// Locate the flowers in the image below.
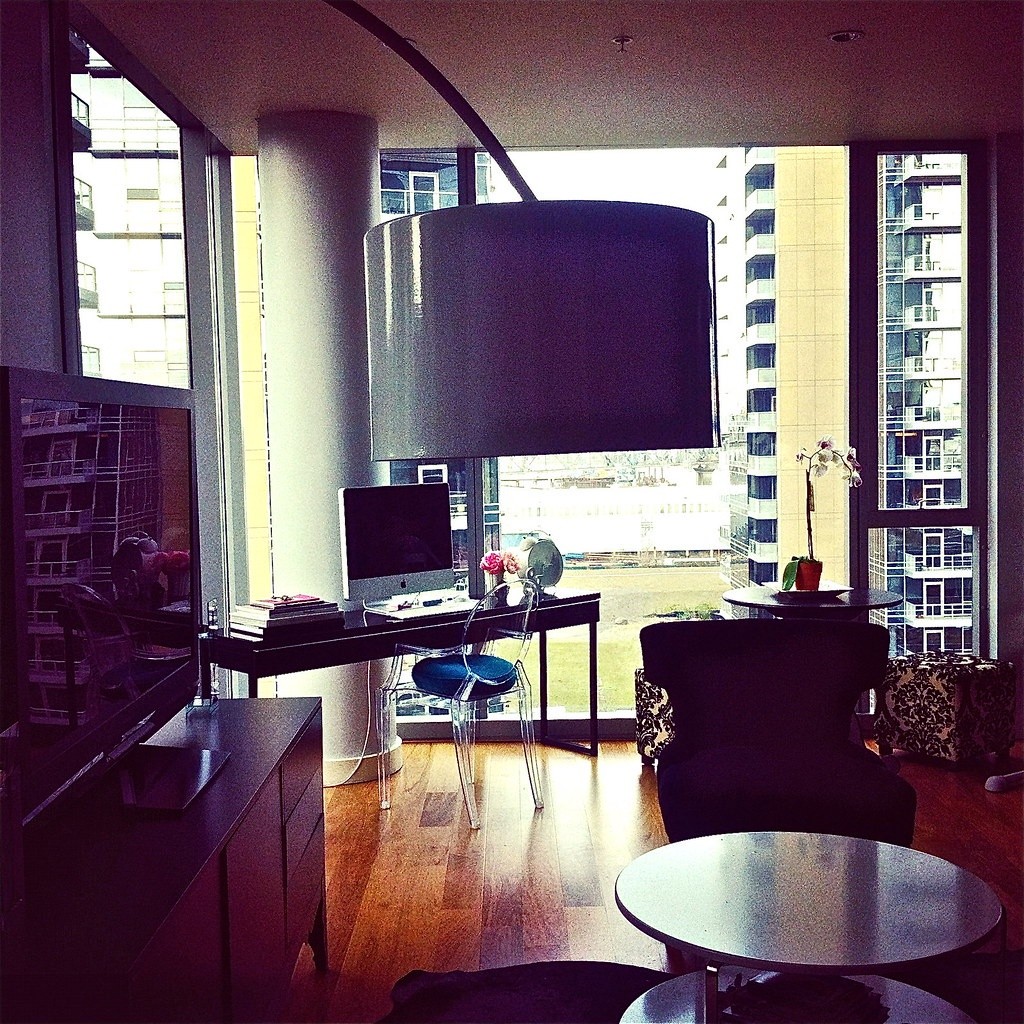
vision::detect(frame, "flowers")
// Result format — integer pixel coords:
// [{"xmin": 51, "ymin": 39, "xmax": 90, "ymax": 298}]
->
[
  {"xmin": 781, "ymin": 435, "xmax": 863, "ymax": 590},
  {"xmin": 479, "ymin": 551, "xmax": 520, "ymax": 575}
]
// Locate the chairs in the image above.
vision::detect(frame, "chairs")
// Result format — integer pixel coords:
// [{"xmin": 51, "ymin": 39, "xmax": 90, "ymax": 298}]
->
[
  {"xmin": 635, "ymin": 617, "xmax": 918, "ymax": 841},
  {"xmin": 373, "ymin": 577, "xmax": 545, "ymax": 831}
]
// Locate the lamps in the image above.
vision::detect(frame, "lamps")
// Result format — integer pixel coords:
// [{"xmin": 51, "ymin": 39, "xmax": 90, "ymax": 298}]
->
[{"xmin": 332, "ymin": 1, "xmax": 720, "ymax": 464}]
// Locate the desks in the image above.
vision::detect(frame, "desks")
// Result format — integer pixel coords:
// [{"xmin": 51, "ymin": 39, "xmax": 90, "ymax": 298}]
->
[
  {"xmin": 722, "ymin": 587, "xmax": 904, "ymax": 618},
  {"xmin": 614, "ymin": 832, "xmax": 1003, "ymax": 1024},
  {"xmin": 198, "ymin": 589, "xmax": 601, "ymax": 756}
]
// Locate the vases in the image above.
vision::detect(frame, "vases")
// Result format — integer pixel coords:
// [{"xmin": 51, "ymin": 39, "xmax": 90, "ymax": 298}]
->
[
  {"xmin": 796, "ymin": 561, "xmax": 822, "ymax": 590},
  {"xmin": 493, "ymin": 575, "xmax": 510, "ymax": 607}
]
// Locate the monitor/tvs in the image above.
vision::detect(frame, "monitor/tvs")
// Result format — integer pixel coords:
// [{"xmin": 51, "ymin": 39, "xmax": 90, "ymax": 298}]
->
[
  {"xmin": 338, "ymin": 483, "xmax": 454, "ymax": 612},
  {"xmin": 0, "ymin": 367, "xmax": 233, "ymax": 829}
]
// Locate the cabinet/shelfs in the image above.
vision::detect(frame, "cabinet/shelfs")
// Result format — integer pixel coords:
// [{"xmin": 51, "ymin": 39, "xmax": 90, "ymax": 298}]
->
[{"xmin": 0, "ymin": 698, "xmax": 327, "ymax": 1024}]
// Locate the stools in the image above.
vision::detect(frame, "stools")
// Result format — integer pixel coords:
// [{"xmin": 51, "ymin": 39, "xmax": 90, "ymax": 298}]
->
[
  {"xmin": 876, "ymin": 651, "xmax": 1017, "ymax": 769},
  {"xmin": 635, "ymin": 669, "xmax": 676, "ymax": 762}
]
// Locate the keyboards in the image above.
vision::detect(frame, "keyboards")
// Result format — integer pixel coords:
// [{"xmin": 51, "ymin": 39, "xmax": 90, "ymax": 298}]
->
[{"xmin": 389, "ymin": 601, "xmax": 483, "ymax": 621}]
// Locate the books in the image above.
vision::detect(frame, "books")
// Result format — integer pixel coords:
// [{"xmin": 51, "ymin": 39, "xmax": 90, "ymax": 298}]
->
[{"xmin": 229, "ymin": 593, "xmax": 346, "ymax": 639}]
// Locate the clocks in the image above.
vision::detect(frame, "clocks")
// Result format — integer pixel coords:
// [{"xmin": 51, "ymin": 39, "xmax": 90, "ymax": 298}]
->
[{"xmin": 518, "ymin": 530, "xmax": 563, "ymax": 588}]
